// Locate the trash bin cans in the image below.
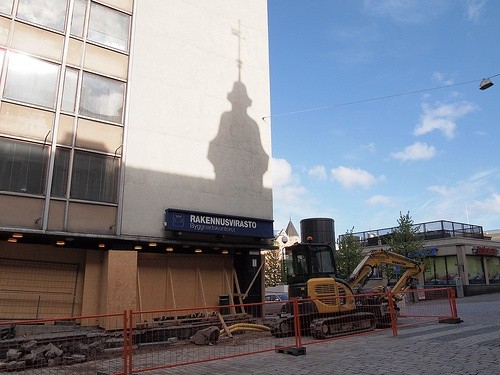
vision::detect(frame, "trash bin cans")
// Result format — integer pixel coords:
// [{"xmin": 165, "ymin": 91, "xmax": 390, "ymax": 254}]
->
[{"xmin": 218, "ymin": 293, "xmax": 249, "ymax": 314}]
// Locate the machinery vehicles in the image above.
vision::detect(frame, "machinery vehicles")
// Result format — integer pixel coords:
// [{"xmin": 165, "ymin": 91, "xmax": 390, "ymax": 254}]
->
[{"xmin": 268, "ymin": 236, "xmax": 425, "ymax": 339}]
